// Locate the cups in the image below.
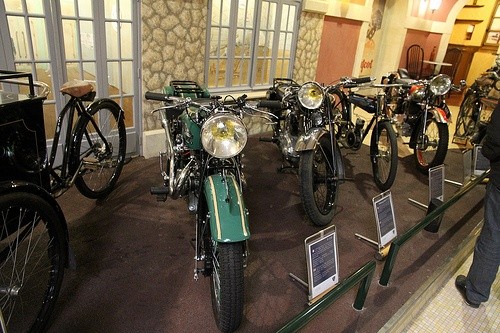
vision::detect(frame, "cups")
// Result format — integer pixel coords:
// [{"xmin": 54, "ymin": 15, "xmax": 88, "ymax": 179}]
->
[{"xmin": 464, "ymin": 24, "xmax": 475, "ymax": 40}]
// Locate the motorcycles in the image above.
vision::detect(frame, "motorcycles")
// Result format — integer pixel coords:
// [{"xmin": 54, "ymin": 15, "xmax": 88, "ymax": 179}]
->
[
  {"xmin": 257, "ymin": 76, "xmax": 376, "ymax": 227},
  {"xmin": 451, "ymin": 52, "xmax": 500, "ymax": 146},
  {"xmin": 144, "ymin": 79, "xmax": 286, "ymax": 333},
  {"xmin": 381, "ymin": 67, "xmax": 467, "ymax": 177}
]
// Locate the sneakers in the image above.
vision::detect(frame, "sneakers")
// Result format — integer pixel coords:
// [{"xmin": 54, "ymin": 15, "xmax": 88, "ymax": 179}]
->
[{"xmin": 455, "ymin": 275, "xmax": 480, "ymax": 308}]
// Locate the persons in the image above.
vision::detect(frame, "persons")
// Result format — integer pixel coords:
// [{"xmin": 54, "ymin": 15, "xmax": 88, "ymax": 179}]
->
[{"xmin": 454, "ymin": 100, "xmax": 500, "ymax": 308}]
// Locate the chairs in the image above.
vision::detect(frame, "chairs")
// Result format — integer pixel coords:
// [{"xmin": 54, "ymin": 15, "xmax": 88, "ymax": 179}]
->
[{"xmin": 406, "ymin": 45, "xmax": 424, "ymax": 80}]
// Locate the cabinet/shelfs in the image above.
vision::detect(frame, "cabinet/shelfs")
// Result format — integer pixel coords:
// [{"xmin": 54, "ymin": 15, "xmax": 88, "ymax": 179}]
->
[
  {"xmin": 455, "ymin": 4, "xmax": 484, "ymax": 22},
  {"xmin": 439, "ymin": 44, "xmax": 482, "ymax": 106}
]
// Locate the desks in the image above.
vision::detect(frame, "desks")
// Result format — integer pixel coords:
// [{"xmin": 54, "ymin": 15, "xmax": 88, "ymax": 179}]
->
[
  {"xmin": 421, "ymin": 59, "xmax": 452, "ymax": 76},
  {"xmin": 0, "ymin": 69, "xmax": 51, "ymax": 241}
]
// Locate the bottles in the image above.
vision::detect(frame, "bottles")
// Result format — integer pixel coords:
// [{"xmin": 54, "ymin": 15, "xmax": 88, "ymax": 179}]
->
[{"xmin": 429, "ymin": 46, "xmax": 437, "ymax": 61}]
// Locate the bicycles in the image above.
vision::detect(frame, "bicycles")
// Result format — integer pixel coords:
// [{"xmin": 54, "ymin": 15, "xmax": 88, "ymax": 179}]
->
[
  {"xmin": 321, "ymin": 74, "xmax": 413, "ymax": 193},
  {"xmin": 0, "ymin": 78, "xmax": 140, "ymax": 333}
]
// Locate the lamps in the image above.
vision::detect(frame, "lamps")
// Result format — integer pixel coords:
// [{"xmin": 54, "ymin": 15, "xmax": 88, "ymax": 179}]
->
[{"xmin": 430, "ymin": 0, "xmax": 442, "ymax": 15}]
